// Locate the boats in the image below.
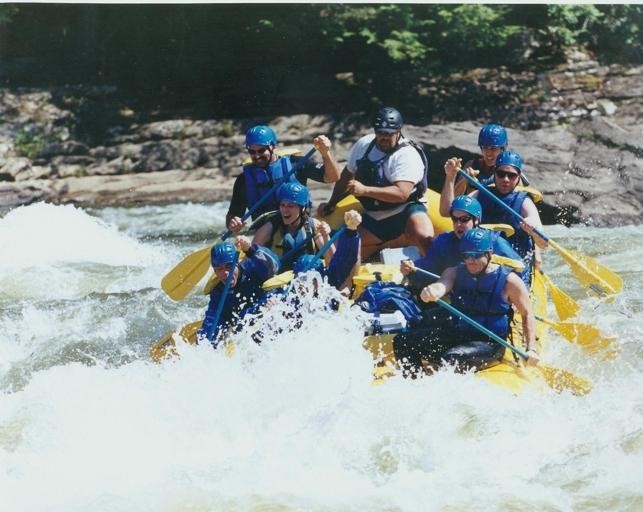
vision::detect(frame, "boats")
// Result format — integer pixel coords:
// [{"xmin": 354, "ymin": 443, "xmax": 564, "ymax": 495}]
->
[{"xmin": 147, "ymin": 186, "xmax": 550, "ymax": 403}]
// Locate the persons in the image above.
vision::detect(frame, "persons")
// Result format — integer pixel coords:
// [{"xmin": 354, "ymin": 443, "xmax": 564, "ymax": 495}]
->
[
  {"xmin": 225, "ymin": 124, "xmax": 342, "ymax": 233},
  {"xmin": 454, "ymin": 121, "xmax": 530, "ymax": 197},
  {"xmin": 317, "ymin": 105, "xmax": 434, "ymax": 262},
  {"xmin": 195, "ymin": 240, "xmax": 282, "ymax": 346},
  {"xmin": 294, "ymin": 209, "xmax": 363, "ymax": 289},
  {"xmin": 393, "ymin": 228, "xmax": 540, "ymax": 377},
  {"xmin": 440, "ymin": 149, "xmax": 550, "ymax": 291},
  {"xmin": 400, "ymin": 195, "xmax": 531, "ymax": 308},
  {"xmin": 251, "ymin": 182, "xmax": 336, "ymax": 269}
]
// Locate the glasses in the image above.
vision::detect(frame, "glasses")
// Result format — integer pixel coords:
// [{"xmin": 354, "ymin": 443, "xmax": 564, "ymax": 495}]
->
[
  {"xmin": 451, "ymin": 216, "xmax": 472, "ymax": 222},
  {"xmin": 462, "ymin": 253, "xmax": 483, "ymax": 259},
  {"xmin": 497, "ymin": 170, "xmax": 517, "ymax": 181},
  {"xmin": 248, "ymin": 148, "xmax": 266, "ymax": 154}
]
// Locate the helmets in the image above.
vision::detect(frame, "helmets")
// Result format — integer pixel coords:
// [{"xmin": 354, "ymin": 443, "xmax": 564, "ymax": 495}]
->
[
  {"xmin": 211, "ymin": 242, "xmax": 235, "ymax": 267},
  {"xmin": 478, "ymin": 124, "xmax": 521, "ymax": 171},
  {"xmin": 293, "ymin": 254, "xmax": 323, "ymax": 276},
  {"xmin": 459, "ymin": 229, "xmax": 493, "ymax": 253},
  {"xmin": 246, "ymin": 125, "xmax": 278, "ymax": 146},
  {"xmin": 372, "ymin": 106, "xmax": 403, "ymax": 133},
  {"xmin": 450, "ymin": 195, "xmax": 482, "ymax": 223},
  {"xmin": 276, "ymin": 182, "xmax": 310, "ymax": 206}
]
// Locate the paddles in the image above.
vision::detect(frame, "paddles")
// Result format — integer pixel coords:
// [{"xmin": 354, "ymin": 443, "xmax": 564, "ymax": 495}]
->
[
  {"xmin": 161, "ymin": 146, "xmax": 320, "ymax": 301},
  {"xmin": 433, "ymin": 296, "xmax": 592, "ymax": 397},
  {"xmin": 151, "ymin": 321, "xmax": 206, "ymax": 367},
  {"xmin": 453, "ymin": 166, "xmax": 624, "ymax": 304},
  {"xmin": 535, "ymin": 261, "xmax": 583, "ymax": 322},
  {"xmin": 533, "ymin": 314, "xmax": 619, "ymax": 364}
]
